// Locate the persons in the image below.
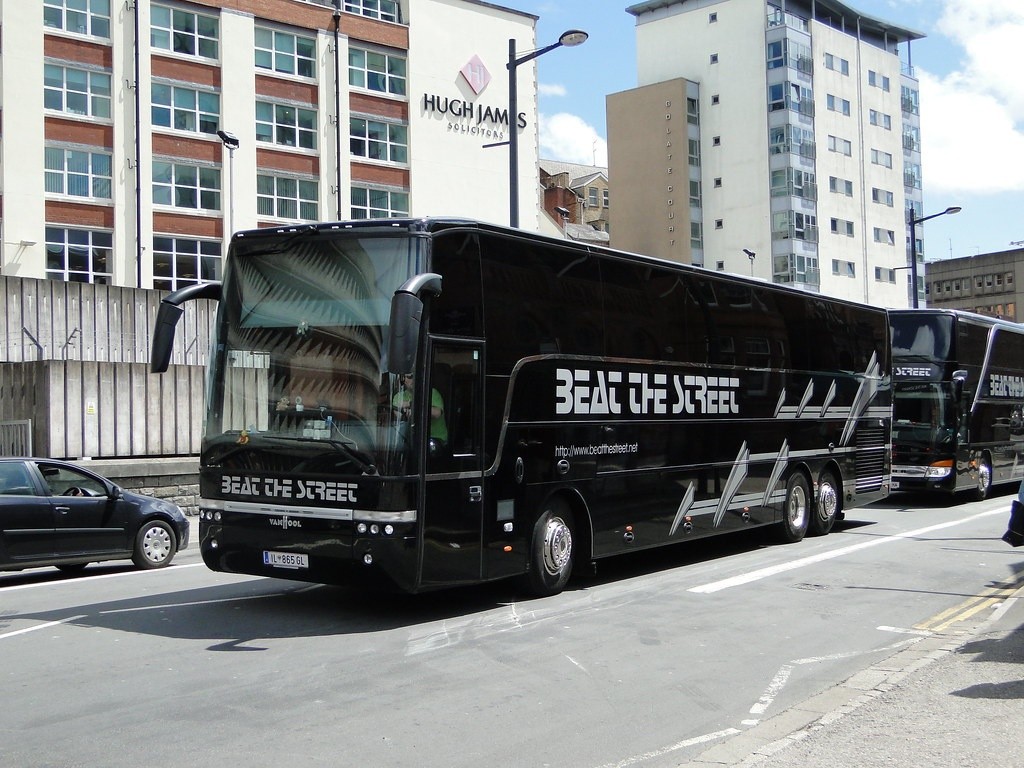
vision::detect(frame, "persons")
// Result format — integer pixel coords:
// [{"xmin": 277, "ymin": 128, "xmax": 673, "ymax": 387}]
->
[{"xmin": 393, "ymin": 372, "xmax": 449, "ymax": 472}]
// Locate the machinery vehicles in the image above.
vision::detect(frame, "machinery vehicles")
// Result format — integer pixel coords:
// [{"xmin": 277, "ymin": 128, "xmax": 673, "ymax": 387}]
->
[{"xmin": 888, "ymin": 309, "xmax": 1024, "ymax": 499}]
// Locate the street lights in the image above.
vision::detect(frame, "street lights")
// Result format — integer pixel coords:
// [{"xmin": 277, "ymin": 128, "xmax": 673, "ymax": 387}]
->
[
  {"xmin": 507, "ymin": 32, "xmax": 586, "ymax": 229},
  {"xmin": 910, "ymin": 207, "xmax": 959, "ymax": 308}
]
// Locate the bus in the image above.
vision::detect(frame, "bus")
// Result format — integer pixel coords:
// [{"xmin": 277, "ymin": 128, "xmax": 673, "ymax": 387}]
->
[{"xmin": 151, "ymin": 217, "xmax": 894, "ymax": 598}]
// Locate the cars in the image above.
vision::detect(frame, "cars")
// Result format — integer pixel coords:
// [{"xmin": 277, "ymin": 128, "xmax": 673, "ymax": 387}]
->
[{"xmin": 1, "ymin": 457, "xmax": 189, "ymax": 571}]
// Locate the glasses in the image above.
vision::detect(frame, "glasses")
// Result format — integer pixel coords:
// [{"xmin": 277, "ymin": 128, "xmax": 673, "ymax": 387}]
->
[{"xmin": 404, "ymin": 374, "xmax": 413, "ymax": 379}]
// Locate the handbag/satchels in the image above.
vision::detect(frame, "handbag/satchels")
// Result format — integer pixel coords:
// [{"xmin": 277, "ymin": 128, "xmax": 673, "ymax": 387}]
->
[{"xmin": 1002, "ymin": 500, "xmax": 1024, "ymax": 547}]
